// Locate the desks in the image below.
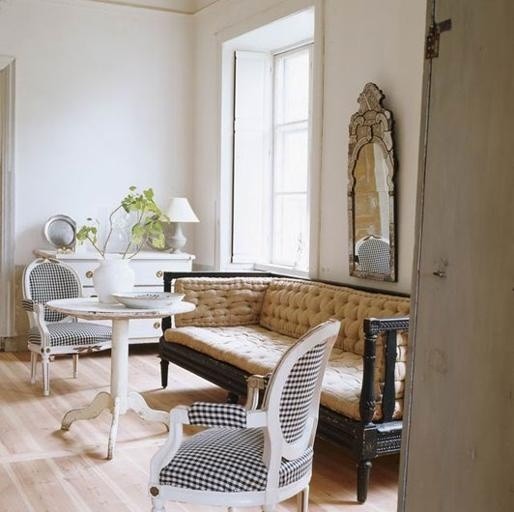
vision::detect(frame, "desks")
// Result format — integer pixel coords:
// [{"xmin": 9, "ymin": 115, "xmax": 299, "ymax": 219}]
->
[{"xmin": 46, "ymin": 297, "xmax": 195, "ymax": 461}]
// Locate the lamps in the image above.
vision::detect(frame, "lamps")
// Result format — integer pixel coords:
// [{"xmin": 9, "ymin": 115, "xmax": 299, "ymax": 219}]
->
[{"xmin": 160, "ymin": 197, "xmax": 200, "ymax": 255}]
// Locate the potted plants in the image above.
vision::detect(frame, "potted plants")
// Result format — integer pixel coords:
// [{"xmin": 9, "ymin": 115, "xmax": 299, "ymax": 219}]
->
[{"xmin": 76, "ymin": 186, "xmax": 170, "ymax": 304}]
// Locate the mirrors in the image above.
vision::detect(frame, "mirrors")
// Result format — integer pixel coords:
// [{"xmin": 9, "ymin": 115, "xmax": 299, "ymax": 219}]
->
[{"xmin": 347, "ymin": 82, "xmax": 398, "ymax": 283}]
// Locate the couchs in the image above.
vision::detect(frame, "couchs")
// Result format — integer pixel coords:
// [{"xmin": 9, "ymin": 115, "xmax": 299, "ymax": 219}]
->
[{"xmin": 158, "ymin": 271, "xmax": 410, "ymax": 505}]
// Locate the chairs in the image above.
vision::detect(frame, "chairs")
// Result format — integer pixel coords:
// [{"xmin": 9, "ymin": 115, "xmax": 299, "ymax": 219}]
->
[
  {"xmin": 21, "ymin": 259, "xmax": 112, "ymax": 397},
  {"xmin": 149, "ymin": 320, "xmax": 342, "ymax": 512},
  {"xmin": 354, "ymin": 234, "xmax": 390, "ymax": 273}
]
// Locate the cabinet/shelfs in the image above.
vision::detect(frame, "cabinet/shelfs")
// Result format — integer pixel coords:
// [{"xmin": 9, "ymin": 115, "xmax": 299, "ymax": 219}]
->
[{"xmin": 34, "ymin": 248, "xmax": 195, "ymax": 344}]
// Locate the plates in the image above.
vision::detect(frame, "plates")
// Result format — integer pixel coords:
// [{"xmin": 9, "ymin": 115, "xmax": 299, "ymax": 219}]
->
[{"xmin": 111, "ymin": 291, "xmax": 186, "ymax": 310}]
